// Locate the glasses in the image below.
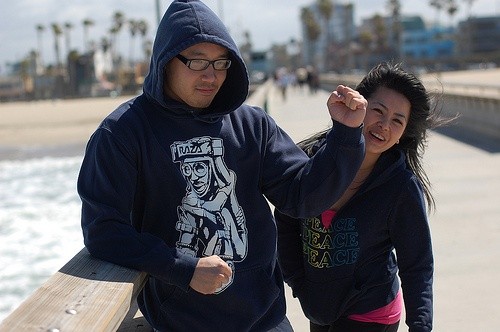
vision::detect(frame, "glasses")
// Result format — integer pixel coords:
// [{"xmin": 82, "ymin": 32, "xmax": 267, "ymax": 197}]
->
[{"xmin": 176, "ymin": 54, "xmax": 232, "ymax": 71}]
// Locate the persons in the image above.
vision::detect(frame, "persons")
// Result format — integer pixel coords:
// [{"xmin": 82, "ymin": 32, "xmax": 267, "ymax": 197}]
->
[
  {"xmin": 275, "ymin": 65, "xmax": 320, "ymax": 99},
  {"xmin": 273, "ymin": 60, "xmax": 463, "ymax": 332},
  {"xmin": 77, "ymin": 0, "xmax": 368, "ymax": 332}
]
